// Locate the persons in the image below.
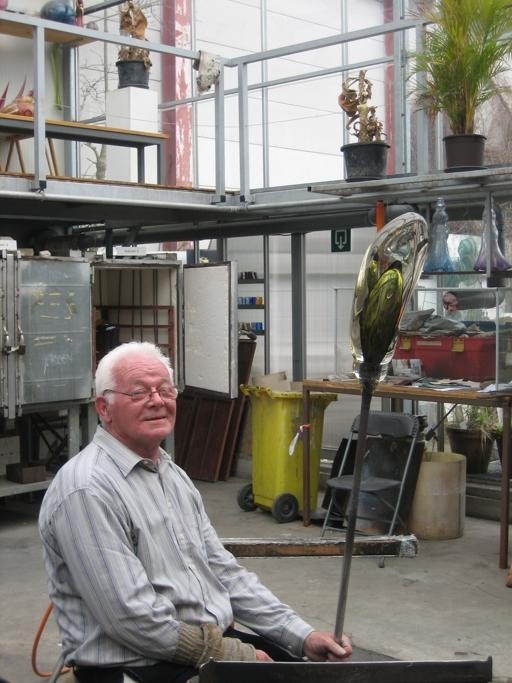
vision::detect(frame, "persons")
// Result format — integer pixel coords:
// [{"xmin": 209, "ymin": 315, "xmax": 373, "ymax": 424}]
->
[{"xmin": 38, "ymin": 341, "xmax": 353, "ymax": 683}]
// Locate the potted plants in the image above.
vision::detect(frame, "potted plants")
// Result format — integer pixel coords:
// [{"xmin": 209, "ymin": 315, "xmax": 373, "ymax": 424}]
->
[
  {"xmin": 403, "ymin": 0, "xmax": 511, "ymax": 173},
  {"xmin": 117, "ymin": 0, "xmax": 152, "ymax": 88},
  {"xmin": 335, "ymin": 69, "xmax": 389, "ymax": 180},
  {"xmin": 445, "ymin": 408, "xmax": 505, "ymax": 475}
]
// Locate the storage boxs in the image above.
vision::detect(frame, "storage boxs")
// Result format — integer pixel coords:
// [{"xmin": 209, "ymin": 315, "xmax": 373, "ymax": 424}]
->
[{"xmin": 391, "ymin": 332, "xmax": 507, "ymax": 385}]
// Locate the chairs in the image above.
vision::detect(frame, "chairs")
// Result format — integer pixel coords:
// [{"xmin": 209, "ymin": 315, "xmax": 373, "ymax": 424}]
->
[{"xmin": 319, "ymin": 411, "xmax": 419, "ymax": 567}]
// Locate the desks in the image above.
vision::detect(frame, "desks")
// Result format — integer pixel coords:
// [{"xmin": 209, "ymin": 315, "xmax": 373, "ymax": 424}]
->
[
  {"xmin": 296, "ymin": 380, "xmax": 512, "ymax": 572},
  {"xmin": 1, "ymin": 111, "xmax": 169, "ymax": 185}
]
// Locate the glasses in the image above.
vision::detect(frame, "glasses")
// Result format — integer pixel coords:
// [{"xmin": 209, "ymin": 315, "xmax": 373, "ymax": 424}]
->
[{"xmin": 103, "ymin": 386, "xmax": 178, "ymax": 402}]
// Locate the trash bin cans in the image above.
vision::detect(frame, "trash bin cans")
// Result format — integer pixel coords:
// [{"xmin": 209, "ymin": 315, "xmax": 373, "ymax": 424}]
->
[{"xmin": 237, "ymin": 384, "xmax": 338, "ymax": 522}]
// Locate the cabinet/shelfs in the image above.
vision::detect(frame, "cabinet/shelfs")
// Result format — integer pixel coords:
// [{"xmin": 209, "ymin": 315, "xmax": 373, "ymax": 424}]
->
[{"xmin": 237, "ymin": 276, "xmax": 266, "ymax": 336}]
